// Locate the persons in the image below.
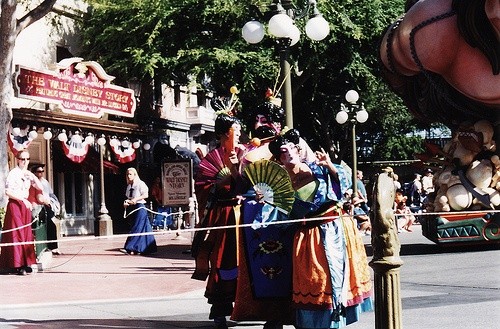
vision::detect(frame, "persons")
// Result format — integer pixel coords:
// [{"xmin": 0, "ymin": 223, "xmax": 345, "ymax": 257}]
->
[
  {"xmin": 191, "ymin": 98, "xmax": 264, "ymax": 329},
  {"xmin": 256, "ymin": 128, "xmax": 376, "ymax": 329},
  {"xmin": 151, "ymin": 177, "xmax": 189, "ymax": 231},
  {"xmin": 119, "ymin": 168, "xmax": 159, "ymax": 255},
  {"xmin": 343, "ymin": 167, "xmax": 435, "ymax": 233},
  {"xmin": 0, "ymin": 149, "xmax": 51, "ymax": 275},
  {"xmin": 230, "ymin": 104, "xmax": 318, "ymax": 329},
  {"xmin": 27, "ymin": 164, "xmax": 65, "ymax": 255}
]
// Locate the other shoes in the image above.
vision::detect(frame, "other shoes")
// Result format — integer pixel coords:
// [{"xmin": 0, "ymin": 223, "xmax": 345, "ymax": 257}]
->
[
  {"xmin": 18, "ymin": 268, "xmax": 27, "ymax": 275},
  {"xmin": 213, "ymin": 316, "xmax": 229, "ymax": 329},
  {"xmin": 120, "ymin": 249, "xmax": 130, "ymax": 255},
  {"xmin": 25, "ymin": 267, "xmax": 32, "ymax": 273}
]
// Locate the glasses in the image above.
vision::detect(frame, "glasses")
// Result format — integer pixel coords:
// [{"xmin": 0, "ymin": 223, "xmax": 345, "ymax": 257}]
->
[
  {"xmin": 18, "ymin": 158, "xmax": 30, "ymax": 160},
  {"xmin": 35, "ymin": 170, "xmax": 44, "ymax": 173}
]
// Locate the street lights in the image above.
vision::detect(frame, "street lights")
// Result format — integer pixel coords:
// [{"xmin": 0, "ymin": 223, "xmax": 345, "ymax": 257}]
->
[
  {"xmin": 335, "ymin": 88, "xmax": 369, "ymax": 197},
  {"xmin": 241, "ymin": 0, "xmax": 331, "ymax": 130}
]
